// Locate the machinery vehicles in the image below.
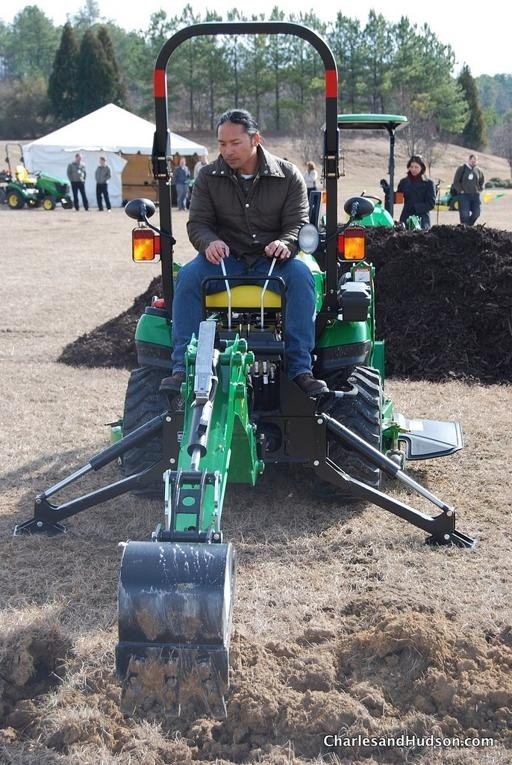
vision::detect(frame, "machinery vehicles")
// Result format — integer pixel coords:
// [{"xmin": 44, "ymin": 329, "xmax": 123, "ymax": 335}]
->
[
  {"xmin": 427, "ymin": 176, "xmax": 461, "ymax": 211},
  {"xmin": 0, "ymin": 170, "xmax": 13, "ymax": 205},
  {"xmin": 10, "ymin": 18, "xmax": 479, "ymax": 722},
  {"xmin": 309, "ymin": 111, "xmax": 423, "ymax": 232}
]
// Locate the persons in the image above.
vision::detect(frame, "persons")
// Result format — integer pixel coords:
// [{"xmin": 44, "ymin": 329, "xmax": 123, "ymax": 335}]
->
[
  {"xmin": 159, "ymin": 109, "xmax": 331, "ymax": 396},
  {"xmin": 397, "ymin": 155, "xmax": 436, "ymax": 232},
  {"xmin": 173, "ymin": 157, "xmax": 191, "ymax": 211},
  {"xmin": 194, "ymin": 155, "xmax": 210, "ymax": 182},
  {"xmin": 94, "ymin": 157, "xmax": 112, "ymax": 213},
  {"xmin": 303, "ymin": 161, "xmax": 316, "ymax": 199},
  {"xmin": 284, "ymin": 158, "xmax": 288, "ymax": 161},
  {"xmin": 67, "ymin": 153, "xmax": 90, "ymax": 211},
  {"xmin": 453, "ymin": 154, "xmax": 484, "ymax": 225}
]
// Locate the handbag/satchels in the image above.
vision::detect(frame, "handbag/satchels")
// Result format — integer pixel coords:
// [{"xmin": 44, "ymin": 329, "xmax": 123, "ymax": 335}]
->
[{"xmin": 449, "ymin": 183, "xmax": 459, "ymax": 197}]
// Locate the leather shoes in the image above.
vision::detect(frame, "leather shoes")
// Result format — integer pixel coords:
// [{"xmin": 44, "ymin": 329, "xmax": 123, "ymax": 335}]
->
[
  {"xmin": 159, "ymin": 371, "xmax": 186, "ymax": 392},
  {"xmin": 294, "ymin": 374, "xmax": 329, "ymax": 396}
]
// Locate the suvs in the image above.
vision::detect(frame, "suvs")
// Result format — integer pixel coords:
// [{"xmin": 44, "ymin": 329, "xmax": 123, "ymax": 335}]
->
[{"xmin": 5, "ymin": 142, "xmax": 73, "ymax": 210}]
